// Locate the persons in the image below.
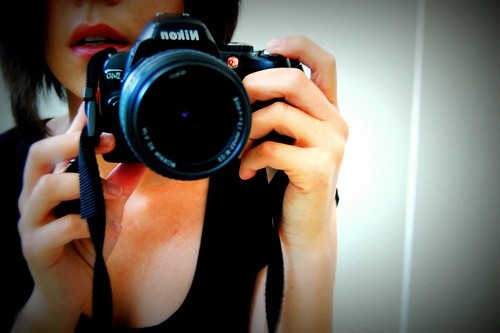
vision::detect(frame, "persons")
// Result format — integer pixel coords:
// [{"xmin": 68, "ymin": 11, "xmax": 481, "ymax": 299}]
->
[{"xmin": 0, "ymin": 0, "xmax": 350, "ymax": 333}]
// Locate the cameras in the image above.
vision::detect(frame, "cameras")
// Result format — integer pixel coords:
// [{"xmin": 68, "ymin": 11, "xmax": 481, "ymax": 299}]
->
[{"xmin": 98, "ymin": 13, "xmax": 301, "ymax": 181}]
